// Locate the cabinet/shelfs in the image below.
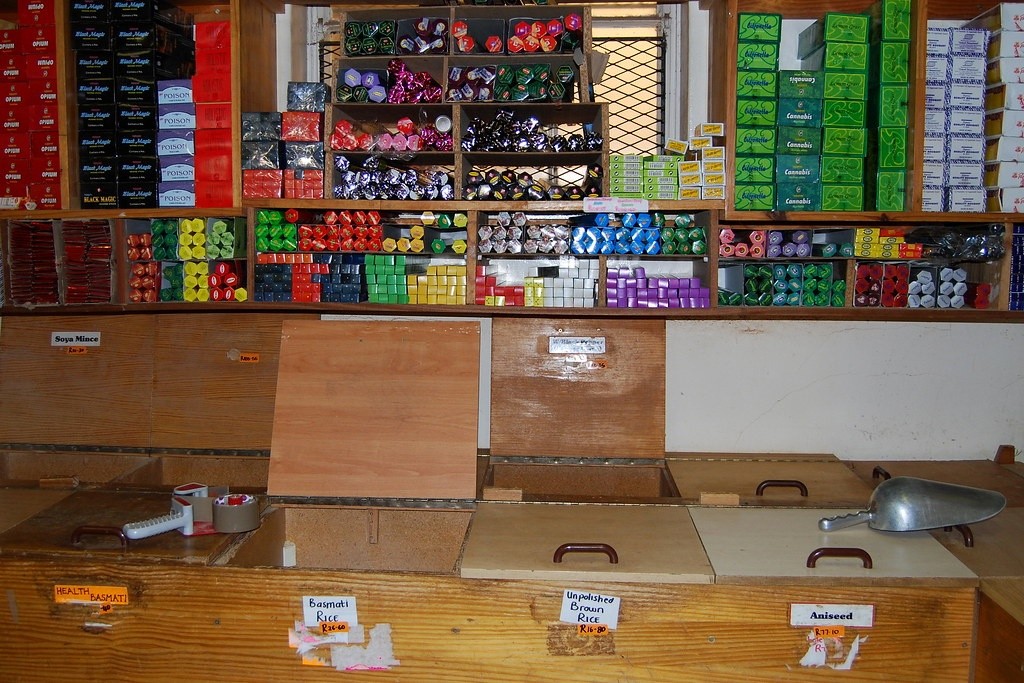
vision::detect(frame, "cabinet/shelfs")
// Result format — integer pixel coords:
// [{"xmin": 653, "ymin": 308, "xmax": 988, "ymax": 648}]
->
[
  {"xmin": 709, "ymin": 0, "xmax": 1024, "ymax": 325},
  {"xmin": 327, "ymin": 6, "xmax": 608, "ymax": 203},
  {"xmin": 240, "ymin": 190, "xmax": 728, "ymax": 319},
  {"xmin": 0, "ymin": 0, "xmax": 245, "ymax": 310}
]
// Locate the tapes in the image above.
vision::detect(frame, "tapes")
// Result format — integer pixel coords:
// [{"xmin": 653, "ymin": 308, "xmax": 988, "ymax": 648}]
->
[{"xmin": 212, "ymin": 494, "xmax": 260, "ymax": 533}]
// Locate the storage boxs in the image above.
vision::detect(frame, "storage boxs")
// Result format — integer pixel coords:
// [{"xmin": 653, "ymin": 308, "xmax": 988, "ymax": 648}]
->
[{"xmin": 1, "ymin": 1, "xmax": 1023, "ymax": 308}]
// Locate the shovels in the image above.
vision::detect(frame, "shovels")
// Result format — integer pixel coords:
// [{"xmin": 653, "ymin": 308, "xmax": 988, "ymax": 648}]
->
[{"xmin": 818, "ymin": 476, "xmax": 1006, "ymax": 532}]
